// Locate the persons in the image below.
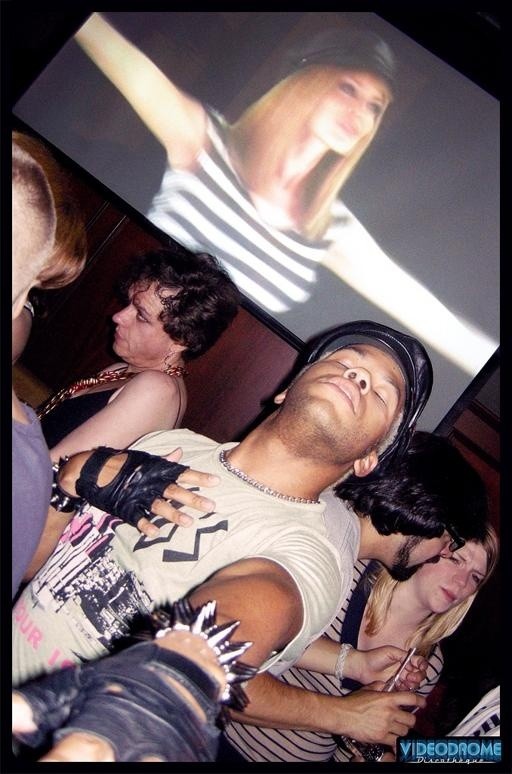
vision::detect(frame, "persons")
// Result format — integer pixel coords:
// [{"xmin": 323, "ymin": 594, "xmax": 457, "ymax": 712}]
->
[
  {"xmin": 9, "ymin": 127, "xmax": 500, "ymax": 762},
  {"xmin": 76, "ymin": 6, "xmax": 500, "ymax": 379}
]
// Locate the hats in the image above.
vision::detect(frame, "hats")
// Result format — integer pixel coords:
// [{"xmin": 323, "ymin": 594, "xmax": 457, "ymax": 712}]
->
[{"xmin": 297, "ymin": 27, "xmax": 402, "ymax": 102}]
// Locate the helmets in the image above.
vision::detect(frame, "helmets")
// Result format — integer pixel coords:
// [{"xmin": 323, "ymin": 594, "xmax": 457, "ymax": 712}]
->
[{"xmin": 307, "ymin": 321, "xmax": 433, "ymax": 463}]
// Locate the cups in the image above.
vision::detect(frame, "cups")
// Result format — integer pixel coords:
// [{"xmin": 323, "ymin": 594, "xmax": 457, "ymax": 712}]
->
[{"xmin": 341, "ymin": 677, "xmax": 419, "ymax": 757}]
